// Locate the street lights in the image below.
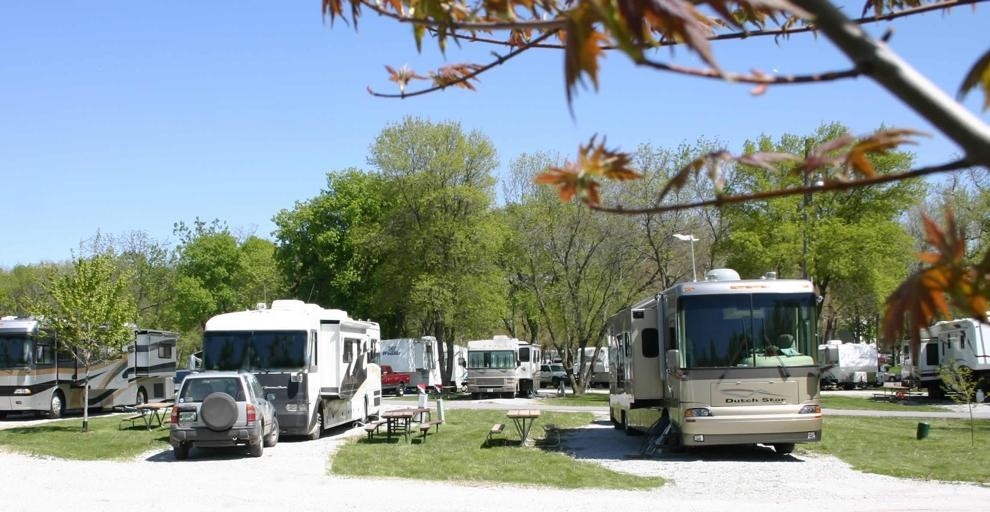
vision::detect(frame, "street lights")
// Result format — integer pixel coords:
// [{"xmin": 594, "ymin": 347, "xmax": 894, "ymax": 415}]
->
[
  {"xmin": 672, "ymin": 230, "xmax": 701, "ymax": 281},
  {"xmin": 802, "ymin": 173, "xmax": 828, "ymax": 277}
]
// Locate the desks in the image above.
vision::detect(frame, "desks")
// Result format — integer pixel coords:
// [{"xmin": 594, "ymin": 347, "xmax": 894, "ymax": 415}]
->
[
  {"xmin": 381, "ymin": 406, "xmax": 434, "ymax": 441},
  {"xmin": 880, "ymin": 382, "xmax": 910, "ymax": 404},
  {"xmin": 133, "ymin": 402, "xmax": 176, "ymax": 432},
  {"xmin": 507, "ymin": 408, "xmax": 541, "ymax": 445}
]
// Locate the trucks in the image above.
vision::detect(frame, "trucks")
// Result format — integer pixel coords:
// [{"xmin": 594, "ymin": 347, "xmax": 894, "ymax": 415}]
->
[{"xmin": 818, "ymin": 313, "xmax": 990, "ymax": 405}]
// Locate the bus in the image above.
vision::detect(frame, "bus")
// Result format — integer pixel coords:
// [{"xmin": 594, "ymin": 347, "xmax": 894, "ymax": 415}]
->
[
  {"xmin": 0, "ymin": 309, "xmax": 186, "ymax": 424},
  {"xmin": 608, "ymin": 268, "xmax": 824, "ymax": 454},
  {"xmin": 381, "ymin": 335, "xmax": 609, "ymax": 397},
  {"xmin": 199, "ymin": 298, "xmax": 381, "ymax": 442}
]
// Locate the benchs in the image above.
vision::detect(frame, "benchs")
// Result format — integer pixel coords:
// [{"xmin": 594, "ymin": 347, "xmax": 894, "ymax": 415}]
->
[
  {"xmin": 542, "ymin": 423, "xmax": 561, "ymax": 445},
  {"xmin": 365, "ymin": 418, "xmax": 394, "ymax": 440},
  {"xmin": 119, "ymin": 413, "xmax": 147, "ymax": 430},
  {"xmin": 873, "ymin": 393, "xmax": 891, "ymax": 400},
  {"xmin": 485, "ymin": 423, "xmax": 506, "ymax": 446},
  {"xmin": 419, "ymin": 419, "xmax": 444, "ymax": 443}
]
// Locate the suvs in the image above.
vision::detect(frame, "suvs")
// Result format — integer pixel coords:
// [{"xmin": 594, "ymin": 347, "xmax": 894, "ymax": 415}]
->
[{"xmin": 168, "ymin": 367, "xmax": 284, "ymax": 459}]
